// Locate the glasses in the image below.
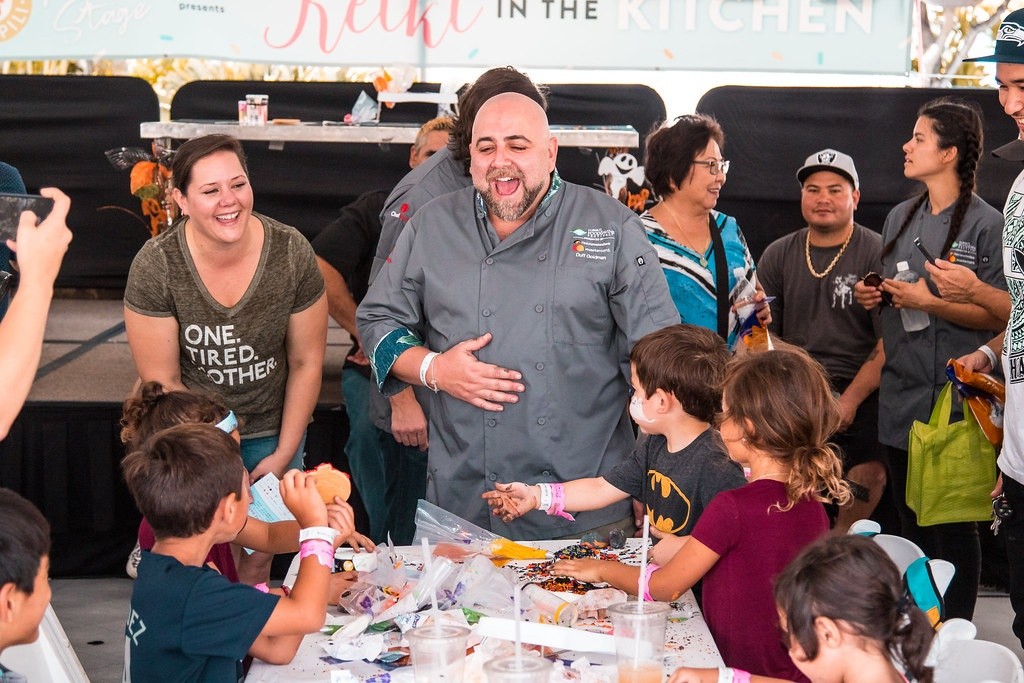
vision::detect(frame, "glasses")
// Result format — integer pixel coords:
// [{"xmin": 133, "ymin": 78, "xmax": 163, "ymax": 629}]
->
[
  {"xmin": 861, "ymin": 270, "xmax": 893, "ymax": 315},
  {"xmin": 691, "ymin": 160, "xmax": 730, "ymax": 175}
]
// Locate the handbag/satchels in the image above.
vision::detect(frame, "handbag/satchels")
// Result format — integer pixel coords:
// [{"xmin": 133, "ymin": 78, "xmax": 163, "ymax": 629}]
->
[{"xmin": 906, "ymin": 380, "xmax": 997, "ymax": 526}]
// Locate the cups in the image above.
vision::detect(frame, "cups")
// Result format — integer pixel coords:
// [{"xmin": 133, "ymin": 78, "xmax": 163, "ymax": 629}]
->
[
  {"xmin": 605, "ymin": 602, "xmax": 672, "ymax": 683},
  {"xmin": 403, "ymin": 624, "xmax": 472, "ymax": 682},
  {"xmin": 483, "ymin": 655, "xmax": 554, "ymax": 683}
]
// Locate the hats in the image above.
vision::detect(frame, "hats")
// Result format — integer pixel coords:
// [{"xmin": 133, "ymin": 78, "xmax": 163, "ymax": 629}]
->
[
  {"xmin": 796, "ymin": 149, "xmax": 859, "ymax": 191},
  {"xmin": 962, "ymin": 8, "xmax": 1024, "ymax": 64}
]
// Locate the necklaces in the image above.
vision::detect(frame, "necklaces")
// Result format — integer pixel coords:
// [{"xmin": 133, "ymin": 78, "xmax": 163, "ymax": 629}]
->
[
  {"xmin": 806, "ymin": 223, "xmax": 854, "ymax": 279},
  {"xmin": 662, "ymin": 198, "xmax": 710, "ymax": 267},
  {"xmin": 750, "ymin": 472, "xmax": 790, "ymax": 483}
]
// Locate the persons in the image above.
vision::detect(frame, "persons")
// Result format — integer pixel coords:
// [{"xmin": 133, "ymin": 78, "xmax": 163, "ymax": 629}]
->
[
  {"xmin": 547, "ymin": 347, "xmax": 855, "ymax": 683},
  {"xmin": 666, "ymin": 529, "xmax": 937, "ymax": 683},
  {"xmin": 120, "ymin": 133, "xmax": 327, "ymax": 590},
  {"xmin": 756, "ymin": 9, "xmax": 1024, "ymax": 651},
  {"xmin": 340, "ymin": 66, "xmax": 682, "ymax": 547},
  {"xmin": 637, "ymin": 110, "xmax": 773, "ymax": 354},
  {"xmin": 120, "ymin": 381, "xmax": 376, "ymax": 604},
  {"xmin": 0, "ymin": 488, "xmax": 52, "ymax": 683},
  {"xmin": 0, "ymin": 160, "xmax": 90, "ymax": 683},
  {"xmin": 307, "ymin": 116, "xmax": 458, "ymax": 546},
  {"xmin": 120, "ymin": 421, "xmax": 355, "ymax": 683},
  {"xmin": 480, "ymin": 324, "xmax": 749, "ymax": 567}
]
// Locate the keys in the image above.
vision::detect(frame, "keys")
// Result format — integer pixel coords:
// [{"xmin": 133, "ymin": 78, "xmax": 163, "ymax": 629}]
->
[{"xmin": 989, "ymin": 494, "xmax": 1012, "ymax": 535}]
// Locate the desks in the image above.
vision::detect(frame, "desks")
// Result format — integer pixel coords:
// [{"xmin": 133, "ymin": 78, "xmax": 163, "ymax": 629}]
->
[
  {"xmin": 244, "ymin": 535, "xmax": 728, "ymax": 683},
  {"xmin": 139, "ymin": 118, "xmax": 639, "ymax": 228}
]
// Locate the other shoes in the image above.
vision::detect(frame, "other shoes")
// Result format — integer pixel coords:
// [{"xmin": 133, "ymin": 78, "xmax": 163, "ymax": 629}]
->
[{"xmin": 126, "ymin": 546, "xmax": 142, "ymax": 579}]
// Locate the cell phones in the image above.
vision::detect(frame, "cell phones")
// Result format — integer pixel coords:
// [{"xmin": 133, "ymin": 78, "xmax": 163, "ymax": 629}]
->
[
  {"xmin": 0, "ymin": 192, "xmax": 54, "ymax": 244},
  {"xmin": 915, "ymin": 236, "xmax": 935, "ymax": 264}
]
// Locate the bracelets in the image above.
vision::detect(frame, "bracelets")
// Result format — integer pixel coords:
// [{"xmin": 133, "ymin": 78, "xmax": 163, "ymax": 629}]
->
[
  {"xmin": 718, "ymin": 667, "xmax": 751, "ymax": 683},
  {"xmin": 419, "ymin": 350, "xmax": 442, "ymax": 393},
  {"xmin": 281, "ymin": 586, "xmax": 289, "ymax": 597},
  {"xmin": 546, "ymin": 481, "xmax": 579, "ymax": 521},
  {"xmin": 300, "ymin": 539, "xmax": 333, "ymax": 569},
  {"xmin": 298, "ymin": 526, "xmax": 334, "ymax": 545},
  {"xmin": 644, "ymin": 564, "xmax": 660, "ymax": 601},
  {"xmin": 535, "ymin": 483, "xmax": 552, "ymax": 510}
]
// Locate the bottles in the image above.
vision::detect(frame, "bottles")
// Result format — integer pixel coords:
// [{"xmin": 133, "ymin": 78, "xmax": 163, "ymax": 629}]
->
[
  {"xmin": 246, "ymin": 94, "xmax": 269, "ymax": 126},
  {"xmin": 518, "ymin": 580, "xmax": 579, "ymax": 626},
  {"xmin": 732, "ymin": 266, "xmax": 773, "ymax": 356},
  {"xmin": 892, "ymin": 261, "xmax": 931, "ymax": 332}
]
